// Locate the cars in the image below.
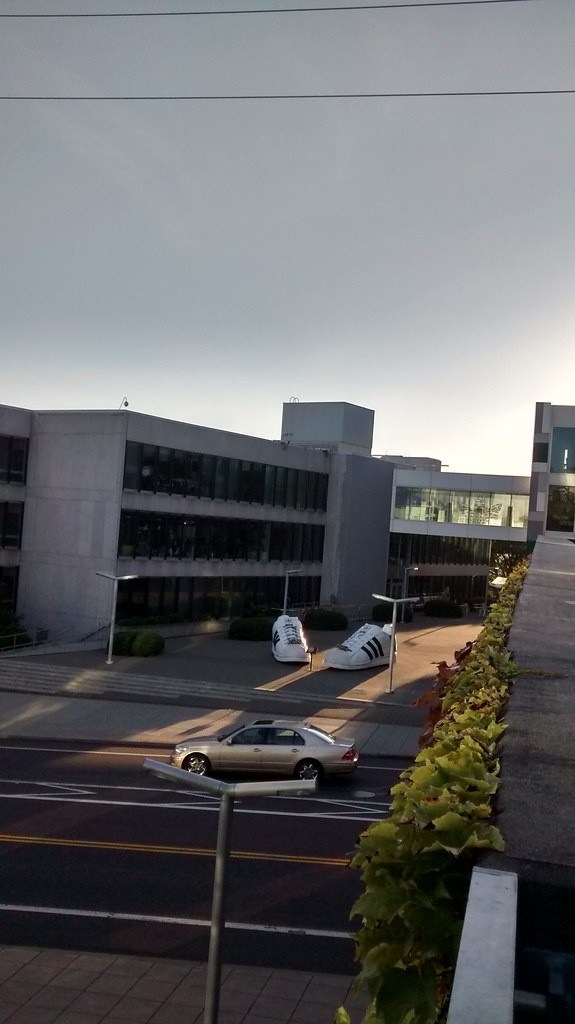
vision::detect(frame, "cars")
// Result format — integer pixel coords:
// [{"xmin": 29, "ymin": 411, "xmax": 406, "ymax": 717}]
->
[{"xmin": 171, "ymin": 719, "xmax": 360, "ymax": 787}]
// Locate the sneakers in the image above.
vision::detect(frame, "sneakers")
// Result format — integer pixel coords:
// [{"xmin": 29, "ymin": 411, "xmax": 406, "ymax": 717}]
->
[
  {"xmin": 322, "ymin": 623, "xmax": 396, "ymax": 669},
  {"xmin": 272, "ymin": 615, "xmax": 311, "ymax": 663}
]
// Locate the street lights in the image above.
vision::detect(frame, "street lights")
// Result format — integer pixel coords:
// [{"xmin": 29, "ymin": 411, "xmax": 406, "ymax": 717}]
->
[
  {"xmin": 371, "ymin": 593, "xmax": 421, "ymax": 694},
  {"xmin": 141, "ymin": 757, "xmax": 317, "ymax": 1024},
  {"xmin": 96, "ymin": 571, "xmax": 139, "ymax": 665},
  {"xmin": 283, "ymin": 568, "xmax": 304, "ymax": 616},
  {"xmin": 400, "ymin": 567, "xmax": 419, "ymax": 623}
]
